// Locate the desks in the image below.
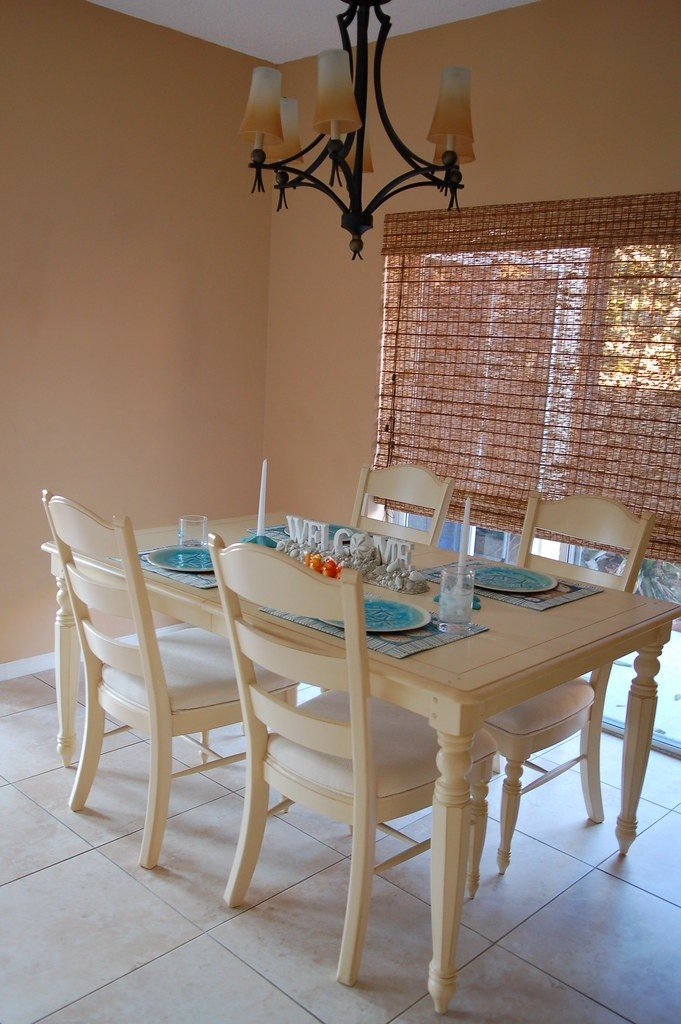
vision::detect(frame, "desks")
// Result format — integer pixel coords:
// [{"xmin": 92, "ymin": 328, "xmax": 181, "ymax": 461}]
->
[{"xmin": 39, "ymin": 513, "xmax": 681, "ymax": 1017}]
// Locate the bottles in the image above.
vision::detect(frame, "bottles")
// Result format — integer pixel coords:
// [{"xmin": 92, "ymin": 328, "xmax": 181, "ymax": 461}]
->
[{"xmin": 177, "ymin": 514, "xmax": 209, "ymax": 564}]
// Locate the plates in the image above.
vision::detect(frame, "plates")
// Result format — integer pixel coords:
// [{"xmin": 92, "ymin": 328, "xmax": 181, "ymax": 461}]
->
[
  {"xmin": 319, "ymin": 594, "xmax": 431, "ymax": 632},
  {"xmin": 284, "ymin": 520, "xmax": 368, "ymax": 545},
  {"xmin": 147, "ymin": 546, "xmax": 216, "ymax": 572},
  {"xmin": 469, "ymin": 566, "xmax": 559, "ymax": 592}
]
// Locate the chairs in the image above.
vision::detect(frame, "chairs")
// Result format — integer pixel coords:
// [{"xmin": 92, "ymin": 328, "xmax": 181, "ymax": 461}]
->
[
  {"xmin": 477, "ymin": 494, "xmax": 653, "ymax": 875},
  {"xmin": 38, "ymin": 489, "xmax": 302, "ymax": 868},
  {"xmin": 204, "ymin": 532, "xmax": 494, "ymax": 987},
  {"xmin": 349, "ymin": 463, "xmax": 458, "ymax": 546}
]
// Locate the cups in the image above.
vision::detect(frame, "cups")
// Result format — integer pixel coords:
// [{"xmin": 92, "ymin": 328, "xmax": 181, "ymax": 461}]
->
[{"xmin": 437, "ymin": 566, "xmax": 474, "ymax": 633}]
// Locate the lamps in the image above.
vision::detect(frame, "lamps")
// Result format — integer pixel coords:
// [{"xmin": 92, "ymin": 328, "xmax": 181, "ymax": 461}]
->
[{"xmin": 240, "ymin": 0, "xmax": 476, "ymax": 261}]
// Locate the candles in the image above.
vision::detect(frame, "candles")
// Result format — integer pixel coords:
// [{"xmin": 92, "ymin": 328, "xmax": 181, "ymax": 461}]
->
[
  {"xmin": 256, "ymin": 459, "xmax": 267, "ymax": 534},
  {"xmin": 454, "ymin": 494, "xmax": 471, "ymax": 585}
]
